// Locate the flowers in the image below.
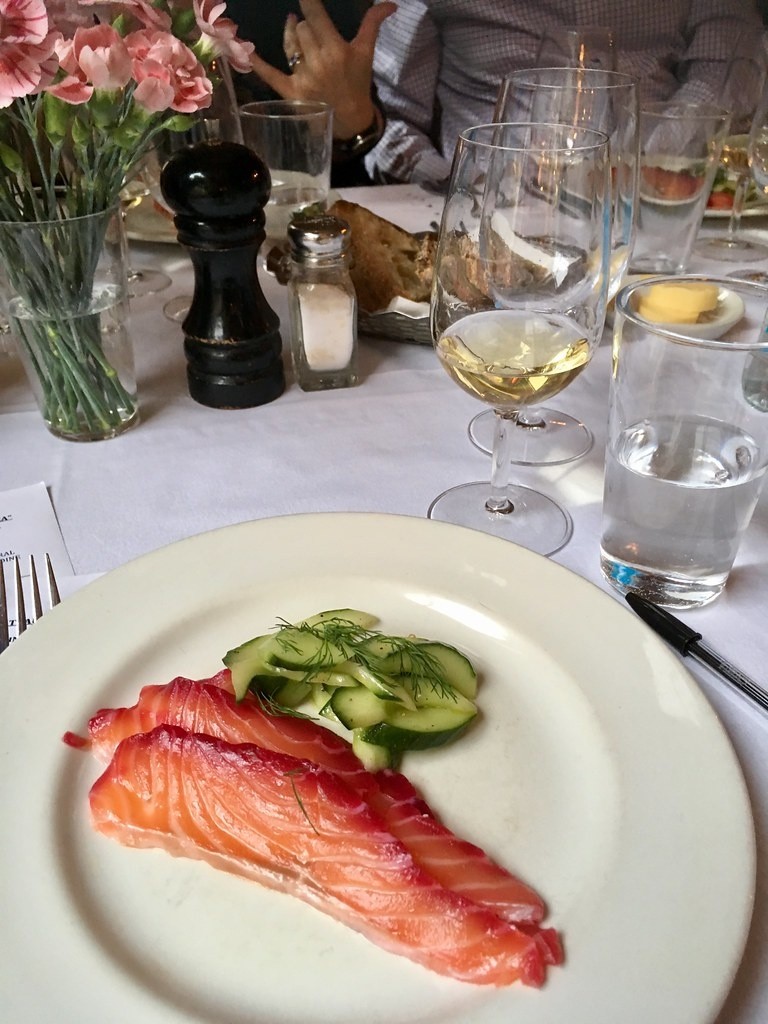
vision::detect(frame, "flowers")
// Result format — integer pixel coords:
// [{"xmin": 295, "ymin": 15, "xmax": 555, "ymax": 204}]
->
[{"xmin": 0, "ymin": 0, "xmax": 257, "ymax": 436}]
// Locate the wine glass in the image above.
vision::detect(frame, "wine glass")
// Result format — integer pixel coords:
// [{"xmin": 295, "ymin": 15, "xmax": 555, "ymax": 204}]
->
[
  {"xmin": 467, "ymin": 67, "xmax": 642, "ymax": 467},
  {"xmin": 427, "ymin": 121, "xmax": 613, "ymax": 558},
  {"xmin": 140, "ymin": 53, "xmax": 245, "ymax": 323},
  {"xmin": 690, "ymin": 23, "xmax": 768, "ymax": 264},
  {"xmin": 122, "ymin": 196, "xmax": 173, "ymax": 301}
]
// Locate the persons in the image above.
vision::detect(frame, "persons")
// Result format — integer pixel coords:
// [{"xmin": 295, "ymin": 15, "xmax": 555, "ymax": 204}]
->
[{"xmin": 219, "ymin": 1, "xmax": 768, "ymax": 191}]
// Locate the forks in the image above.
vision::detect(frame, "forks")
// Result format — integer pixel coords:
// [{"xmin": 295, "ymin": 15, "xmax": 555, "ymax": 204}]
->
[{"xmin": 0, "ymin": 552, "xmax": 63, "ymax": 655}]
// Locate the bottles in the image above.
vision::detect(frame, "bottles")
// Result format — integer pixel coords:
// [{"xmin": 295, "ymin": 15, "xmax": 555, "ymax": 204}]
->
[{"xmin": 286, "ymin": 216, "xmax": 359, "ymax": 393}]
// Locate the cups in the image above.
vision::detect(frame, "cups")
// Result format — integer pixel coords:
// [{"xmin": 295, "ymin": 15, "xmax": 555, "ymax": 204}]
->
[
  {"xmin": 625, "ymin": 100, "xmax": 734, "ymax": 276},
  {"xmin": 598, "ymin": 274, "xmax": 768, "ymax": 612},
  {"xmin": 237, "ymin": 98, "xmax": 335, "ymax": 276},
  {"xmin": 533, "ymin": 23, "xmax": 617, "ymax": 72}
]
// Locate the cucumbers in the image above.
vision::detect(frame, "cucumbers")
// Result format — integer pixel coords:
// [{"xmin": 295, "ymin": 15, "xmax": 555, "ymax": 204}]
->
[
  {"xmin": 221, "ymin": 607, "xmax": 480, "ymax": 772},
  {"xmin": 687, "ymin": 163, "xmax": 759, "ymax": 204}
]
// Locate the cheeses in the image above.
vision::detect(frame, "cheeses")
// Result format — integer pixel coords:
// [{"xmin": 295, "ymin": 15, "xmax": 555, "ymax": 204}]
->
[{"xmin": 632, "ymin": 275, "xmax": 720, "ymax": 324}]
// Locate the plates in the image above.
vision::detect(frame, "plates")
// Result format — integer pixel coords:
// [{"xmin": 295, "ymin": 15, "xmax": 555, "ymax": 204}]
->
[
  {"xmin": 528, "ymin": 153, "xmax": 768, "ymax": 218},
  {"xmin": 123, "ymin": 169, "xmax": 342, "ymax": 241},
  {"xmin": 0, "ymin": 511, "xmax": 768, "ymax": 1024}
]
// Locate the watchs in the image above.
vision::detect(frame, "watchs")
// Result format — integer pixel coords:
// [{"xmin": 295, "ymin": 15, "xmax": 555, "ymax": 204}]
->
[{"xmin": 336, "ymin": 111, "xmax": 379, "ymax": 152}]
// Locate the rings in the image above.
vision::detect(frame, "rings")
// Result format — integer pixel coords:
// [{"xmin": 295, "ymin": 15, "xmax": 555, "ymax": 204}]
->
[{"xmin": 288, "ymin": 52, "xmax": 303, "ymax": 66}]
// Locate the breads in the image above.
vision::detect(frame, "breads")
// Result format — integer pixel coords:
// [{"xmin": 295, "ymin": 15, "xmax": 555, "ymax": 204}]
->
[{"xmin": 324, "ymin": 202, "xmax": 543, "ymax": 303}]
